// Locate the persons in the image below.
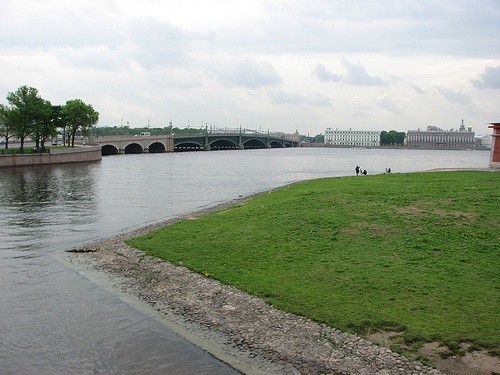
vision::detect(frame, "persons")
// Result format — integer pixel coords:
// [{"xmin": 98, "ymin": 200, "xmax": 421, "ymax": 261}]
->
[
  {"xmin": 356, "ymin": 165, "xmax": 361, "ymax": 176},
  {"xmin": 360, "ymin": 169, "xmax": 363, "ymax": 176}
]
